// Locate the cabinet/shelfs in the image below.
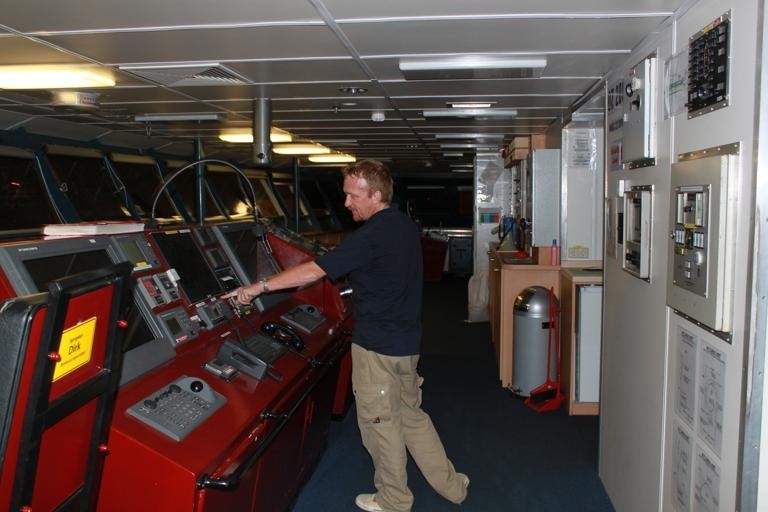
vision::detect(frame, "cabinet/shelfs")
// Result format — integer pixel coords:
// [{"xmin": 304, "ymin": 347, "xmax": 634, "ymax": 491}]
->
[{"xmin": 487, "ymin": 148, "xmax": 560, "ymax": 389}]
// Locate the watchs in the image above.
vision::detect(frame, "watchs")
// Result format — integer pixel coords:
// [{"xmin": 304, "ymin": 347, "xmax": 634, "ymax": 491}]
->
[{"xmin": 259, "ymin": 276, "xmax": 271, "ymax": 295}]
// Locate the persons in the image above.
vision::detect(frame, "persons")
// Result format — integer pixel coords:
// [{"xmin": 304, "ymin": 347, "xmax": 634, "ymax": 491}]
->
[{"xmin": 217, "ymin": 158, "xmax": 471, "ymax": 512}]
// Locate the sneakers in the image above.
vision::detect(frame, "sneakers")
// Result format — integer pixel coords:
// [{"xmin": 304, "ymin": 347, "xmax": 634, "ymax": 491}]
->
[
  {"xmin": 452, "ymin": 472, "xmax": 470, "ymax": 487},
  {"xmin": 354, "ymin": 493, "xmax": 385, "ymax": 511}
]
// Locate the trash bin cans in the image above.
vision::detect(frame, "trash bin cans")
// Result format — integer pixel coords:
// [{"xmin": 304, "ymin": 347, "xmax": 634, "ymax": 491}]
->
[
  {"xmin": 508, "ymin": 285, "xmax": 559, "ymax": 396},
  {"xmin": 423, "ymin": 235, "xmax": 472, "ymax": 280}
]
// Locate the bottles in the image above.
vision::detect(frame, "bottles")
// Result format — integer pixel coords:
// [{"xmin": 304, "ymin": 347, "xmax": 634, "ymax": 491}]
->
[{"xmin": 550, "ymin": 237, "xmax": 557, "ymax": 266}]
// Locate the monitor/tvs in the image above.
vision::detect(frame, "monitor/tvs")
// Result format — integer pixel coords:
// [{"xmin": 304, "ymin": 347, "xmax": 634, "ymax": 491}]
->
[
  {"xmin": 149, "ymin": 228, "xmax": 225, "ymax": 305},
  {"xmin": 0, "ymin": 234, "xmax": 177, "ymax": 388},
  {"xmin": 211, "ymin": 222, "xmax": 295, "ymax": 313}
]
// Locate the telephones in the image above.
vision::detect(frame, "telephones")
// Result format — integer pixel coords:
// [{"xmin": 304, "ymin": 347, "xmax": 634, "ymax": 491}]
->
[{"xmin": 261, "ymin": 322, "xmax": 304, "ymax": 352}]
